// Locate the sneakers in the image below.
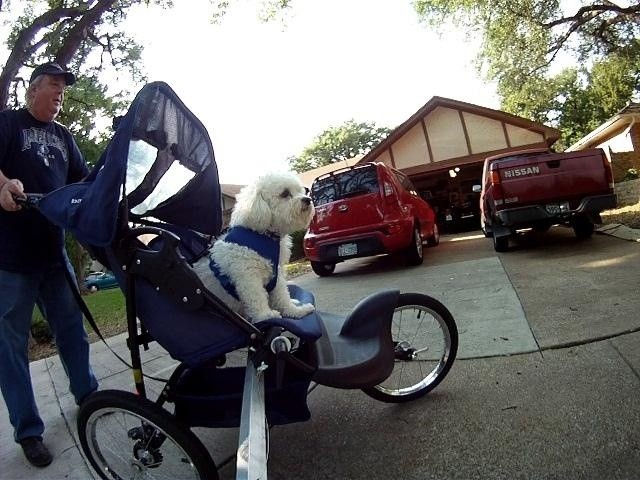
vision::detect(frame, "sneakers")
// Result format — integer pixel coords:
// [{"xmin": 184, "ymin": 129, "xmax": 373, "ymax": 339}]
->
[{"xmin": 18, "ymin": 433, "xmax": 52, "ymax": 467}]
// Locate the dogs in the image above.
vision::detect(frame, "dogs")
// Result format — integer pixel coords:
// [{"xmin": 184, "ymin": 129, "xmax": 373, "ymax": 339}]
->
[{"xmin": 186, "ymin": 166, "xmax": 316, "ymax": 326}]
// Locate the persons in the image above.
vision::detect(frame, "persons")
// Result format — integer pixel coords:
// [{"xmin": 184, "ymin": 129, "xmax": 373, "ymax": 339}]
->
[{"xmin": 0, "ymin": 60, "xmax": 115, "ymax": 469}]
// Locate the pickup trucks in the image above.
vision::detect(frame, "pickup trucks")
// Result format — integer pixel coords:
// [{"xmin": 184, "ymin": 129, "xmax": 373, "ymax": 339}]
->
[{"xmin": 472, "ymin": 148, "xmax": 618, "ymax": 252}]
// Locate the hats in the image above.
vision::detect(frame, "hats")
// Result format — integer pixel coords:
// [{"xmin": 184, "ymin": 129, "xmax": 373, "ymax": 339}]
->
[{"xmin": 27, "ymin": 64, "xmax": 77, "ymax": 86}]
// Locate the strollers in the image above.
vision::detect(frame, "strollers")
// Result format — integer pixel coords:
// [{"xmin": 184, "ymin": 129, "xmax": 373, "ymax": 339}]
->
[{"xmin": 12, "ymin": 80, "xmax": 458, "ymax": 480}]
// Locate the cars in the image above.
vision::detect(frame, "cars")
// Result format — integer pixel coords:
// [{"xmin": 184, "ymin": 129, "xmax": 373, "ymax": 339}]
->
[
  {"xmin": 81, "ymin": 271, "xmax": 118, "ymax": 293},
  {"xmin": 303, "ymin": 161, "xmax": 438, "ymax": 276}
]
